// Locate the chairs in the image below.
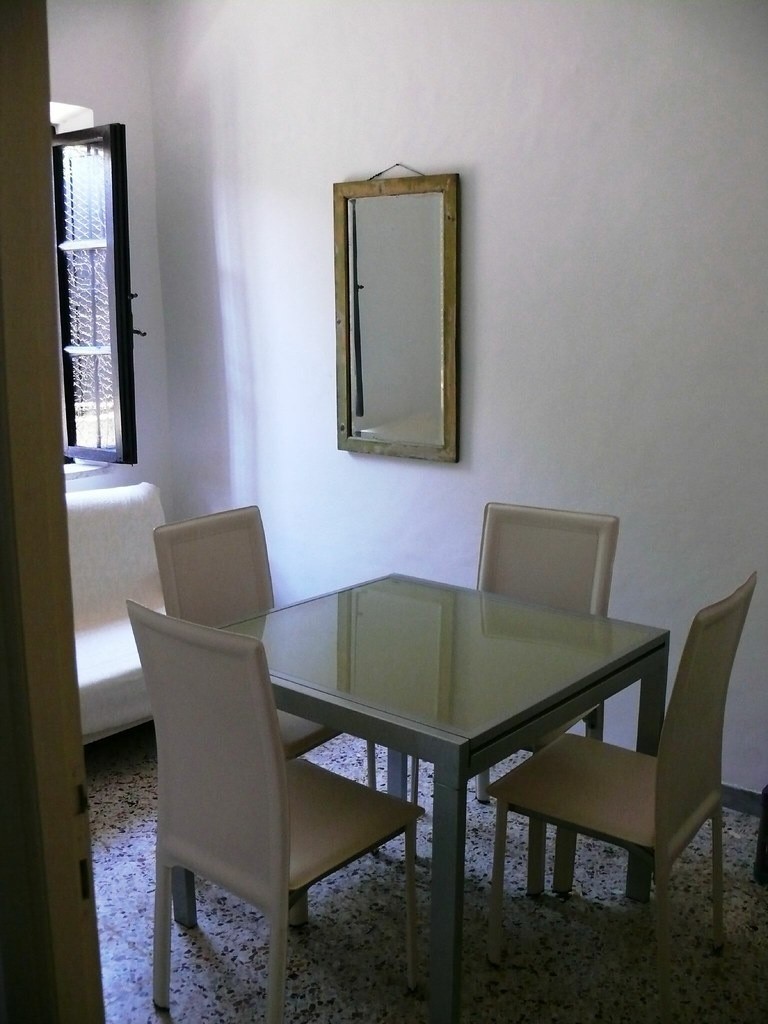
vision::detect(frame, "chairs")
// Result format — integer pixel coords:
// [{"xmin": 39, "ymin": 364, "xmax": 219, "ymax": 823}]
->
[
  {"xmin": 489, "ymin": 573, "xmax": 759, "ymax": 1016},
  {"xmin": 153, "ymin": 505, "xmax": 380, "ymax": 858},
  {"xmin": 125, "ymin": 601, "xmax": 426, "ymax": 1024},
  {"xmin": 476, "ymin": 504, "xmax": 621, "ymax": 804}
]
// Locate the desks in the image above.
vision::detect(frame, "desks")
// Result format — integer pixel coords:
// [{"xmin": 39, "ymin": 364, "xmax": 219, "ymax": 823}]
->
[{"xmin": 171, "ymin": 572, "xmax": 672, "ymax": 1024}]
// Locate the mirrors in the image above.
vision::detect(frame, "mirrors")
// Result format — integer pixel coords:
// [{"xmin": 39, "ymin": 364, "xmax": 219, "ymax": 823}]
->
[{"xmin": 332, "ymin": 173, "xmax": 459, "ymax": 464}]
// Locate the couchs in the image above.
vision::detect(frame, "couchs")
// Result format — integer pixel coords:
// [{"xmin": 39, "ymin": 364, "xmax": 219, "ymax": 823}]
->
[{"xmin": 66, "ymin": 483, "xmax": 168, "ymax": 747}]
